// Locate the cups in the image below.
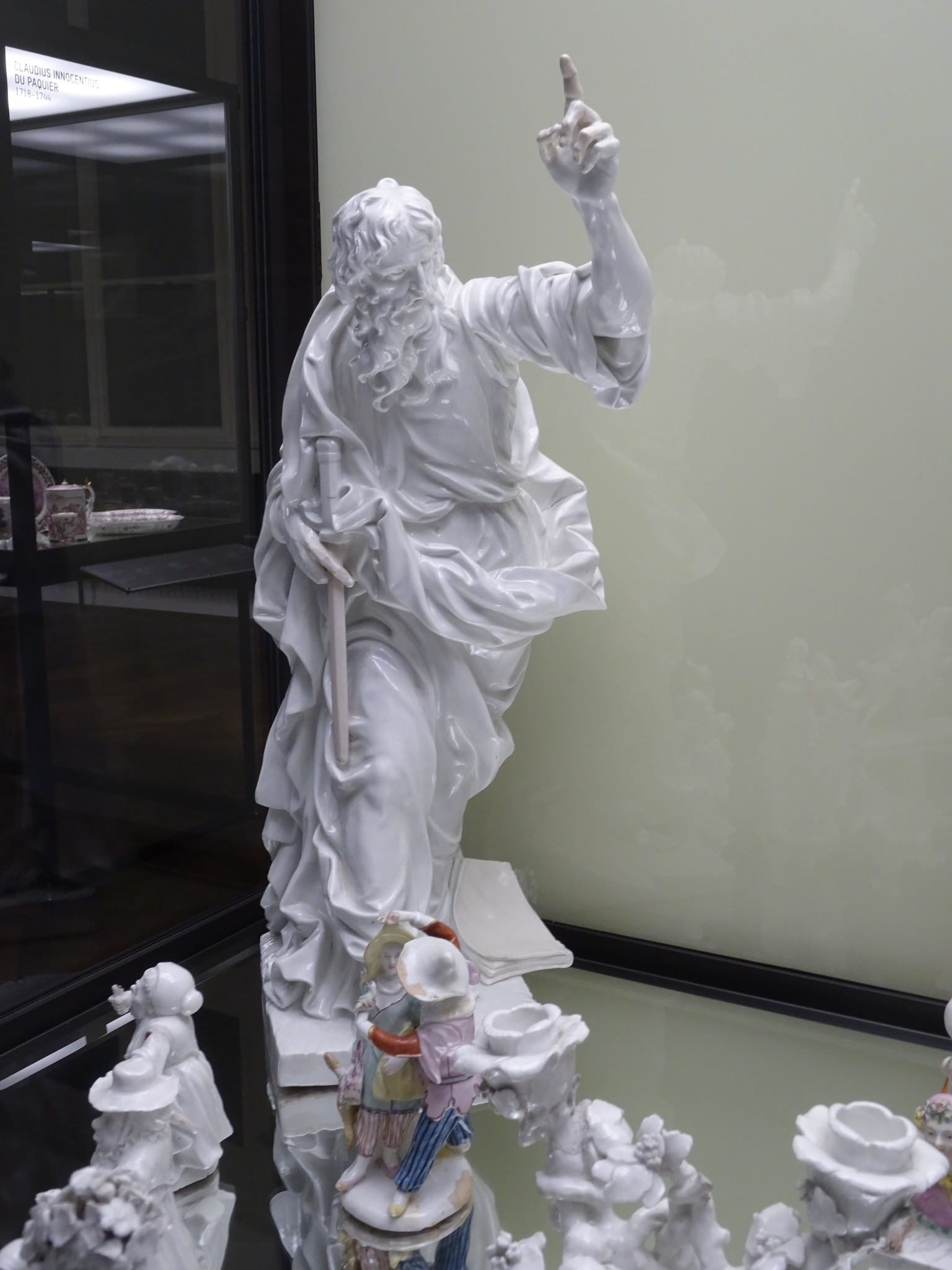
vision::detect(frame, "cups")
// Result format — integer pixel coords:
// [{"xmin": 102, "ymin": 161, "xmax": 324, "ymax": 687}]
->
[
  {"xmin": 51, "ymin": 511, "xmax": 79, "ymax": 545},
  {"xmin": 0, "ymin": 496, "xmax": 12, "ymax": 541},
  {"xmin": 46, "ymin": 479, "xmax": 96, "ymax": 539}
]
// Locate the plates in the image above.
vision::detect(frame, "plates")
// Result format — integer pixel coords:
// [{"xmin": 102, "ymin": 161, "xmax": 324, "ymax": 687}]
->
[
  {"xmin": 0, "ymin": 453, "xmax": 56, "ymax": 533},
  {"xmin": 88, "ymin": 509, "xmax": 185, "ymax": 535}
]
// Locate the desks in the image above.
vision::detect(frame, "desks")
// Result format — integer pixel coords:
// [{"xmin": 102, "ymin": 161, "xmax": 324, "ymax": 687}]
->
[{"xmin": 1, "ymin": 518, "xmax": 257, "ymax": 897}]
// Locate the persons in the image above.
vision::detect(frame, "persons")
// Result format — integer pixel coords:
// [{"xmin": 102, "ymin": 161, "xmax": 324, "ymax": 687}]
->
[
  {"xmin": 334, "ymin": 908, "xmax": 482, "ymax": 1219},
  {"xmin": 249, "ymin": 51, "xmax": 656, "ymax": 1020},
  {"xmin": 911, "ymin": 1094, "xmax": 952, "ymax": 1234},
  {"xmin": 89, "ymin": 961, "xmax": 236, "ymax": 1270}
]
